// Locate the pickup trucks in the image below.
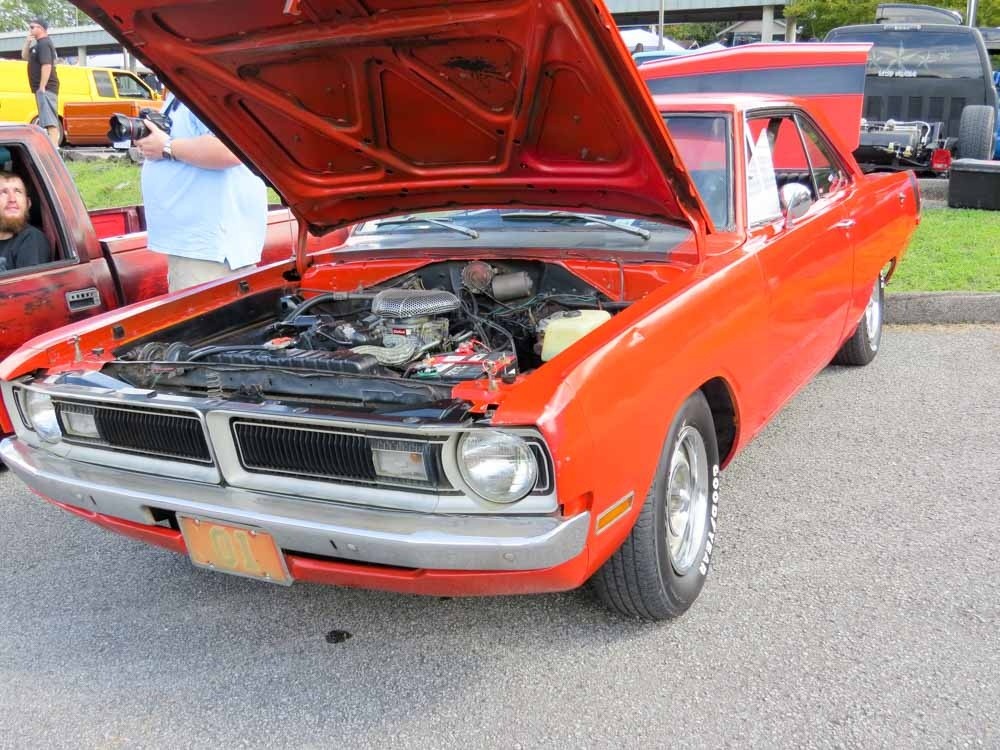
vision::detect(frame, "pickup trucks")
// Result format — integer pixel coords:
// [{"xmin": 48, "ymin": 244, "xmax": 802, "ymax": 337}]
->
[
  {"xmin": 0, "ymin": 122, "xmax": 358, "ymax": 488},
  {"xmin": 0, "ymin": 56, "xmax": 165, "ymax": 156}
]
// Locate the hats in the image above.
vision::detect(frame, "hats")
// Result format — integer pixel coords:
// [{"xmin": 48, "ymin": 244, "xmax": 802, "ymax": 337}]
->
[{"xmin": 28, "ymin": 17, "xmax": 49, "ymax": 29}]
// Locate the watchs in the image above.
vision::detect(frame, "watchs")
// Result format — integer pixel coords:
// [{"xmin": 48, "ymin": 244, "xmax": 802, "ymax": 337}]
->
[{"xmin": 162, "ymin": 138, "xmax": 177, "ymax": 161}]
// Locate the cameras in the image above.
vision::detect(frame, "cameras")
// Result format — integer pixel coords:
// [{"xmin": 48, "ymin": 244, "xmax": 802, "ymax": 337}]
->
[{"xmin": 107, "ymin": 109, "xmax": 172, "ymax": 142}]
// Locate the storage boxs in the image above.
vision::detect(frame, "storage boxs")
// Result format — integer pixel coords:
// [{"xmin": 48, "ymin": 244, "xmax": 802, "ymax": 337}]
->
[{"xmin": 949, "ymin": 158, "xmax": 1000, "ymax": 211}]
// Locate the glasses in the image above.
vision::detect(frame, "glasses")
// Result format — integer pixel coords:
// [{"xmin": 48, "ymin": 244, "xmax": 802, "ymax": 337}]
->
[{"xmin": 30, "ymin": 26, "xmax": 41, "ymax": 29}]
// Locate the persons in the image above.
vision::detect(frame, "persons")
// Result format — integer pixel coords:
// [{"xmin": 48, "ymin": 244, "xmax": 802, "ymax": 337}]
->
[
  {"xmin": 0, "ymin": 169, "xmax": 51, "ymax": 272},
  {"xmin": 21, "ymin": 18, "xmax": 60, "ymax": 149},
  {"xmin": 128, "ymin": 90, "xmax": 269, "ymax": 299}
]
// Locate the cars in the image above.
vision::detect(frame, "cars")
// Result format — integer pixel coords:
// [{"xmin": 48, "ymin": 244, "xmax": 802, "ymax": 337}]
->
[
  {"xmin": 0, "ymin": 0, "xmax": 923, "ymax": 627},
  {"xmin": 612, "ymin": 4, "xmax": 1000, "ymax": 180}
]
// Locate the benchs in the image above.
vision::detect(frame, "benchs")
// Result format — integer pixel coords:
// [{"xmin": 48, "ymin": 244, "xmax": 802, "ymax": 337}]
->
[{"xmin": 683, "ymin": 164, "xmax": 831, "ymax": 225}]
